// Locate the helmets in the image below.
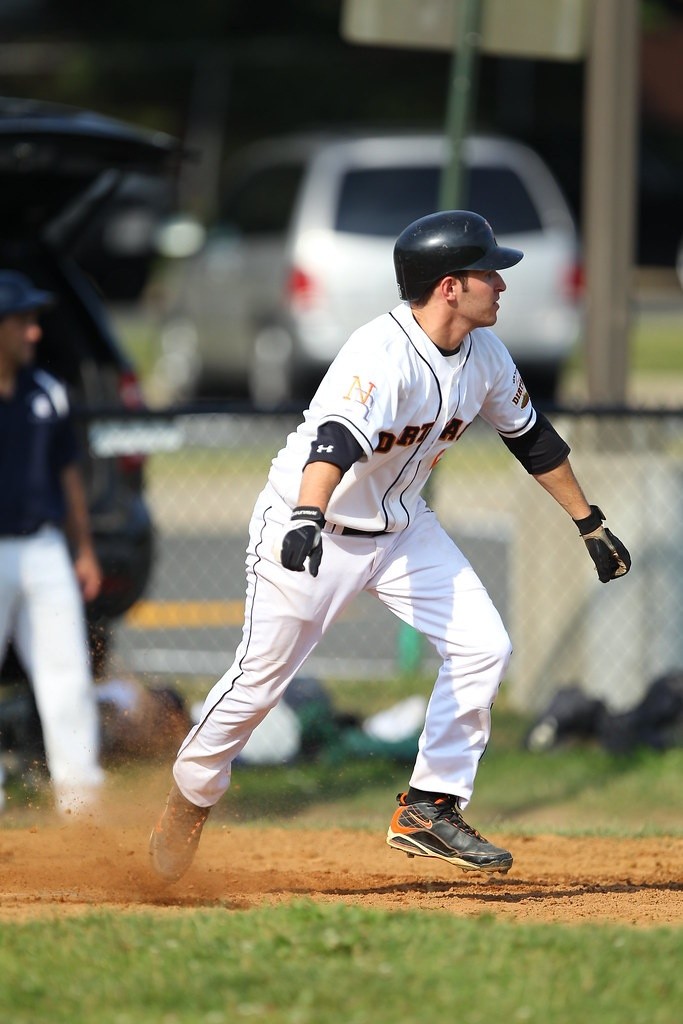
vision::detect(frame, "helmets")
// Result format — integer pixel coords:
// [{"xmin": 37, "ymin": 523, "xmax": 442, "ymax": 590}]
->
[{"xmin": 392, "ymin": 210, "xmax": 525, "ymax": 302}]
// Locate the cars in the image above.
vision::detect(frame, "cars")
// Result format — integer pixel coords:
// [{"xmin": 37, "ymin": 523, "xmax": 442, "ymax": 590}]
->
[{"xmin": 0, "ymin": 91, "xmax": 183, "ymax": 691}]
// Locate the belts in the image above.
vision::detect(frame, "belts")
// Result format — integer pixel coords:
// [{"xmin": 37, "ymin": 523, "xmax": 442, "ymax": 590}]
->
[{"xmin": 322, "ymin": 518, "xmax": 394, "ymax": 538}]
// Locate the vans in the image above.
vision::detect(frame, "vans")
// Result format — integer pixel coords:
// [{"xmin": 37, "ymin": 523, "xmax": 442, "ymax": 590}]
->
[{"xmin": 164, "ymin": 128, "xmax": 584, "ymax": 414}]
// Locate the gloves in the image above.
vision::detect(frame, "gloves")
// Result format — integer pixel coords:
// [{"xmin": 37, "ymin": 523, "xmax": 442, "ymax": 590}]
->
[
  {"xmin": 281, "ymin": 505, "xmax": 325, "ymax": 577},
  {"xmin": 571, "ymin": 505, "xmax": 632, "ymax": 584}
]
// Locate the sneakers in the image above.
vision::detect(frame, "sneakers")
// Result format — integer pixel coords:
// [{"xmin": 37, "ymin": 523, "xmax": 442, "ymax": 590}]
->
[
  {"xmin": 148, "ymin": 779, "xmax": 212, "ymax": 884},
  {"xmin": 385, "ymin": 789, "xmax": 514, "ymax": 875}
]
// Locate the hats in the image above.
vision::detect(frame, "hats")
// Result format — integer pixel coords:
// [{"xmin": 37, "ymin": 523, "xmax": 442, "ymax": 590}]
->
[{"xmin": 1, "ymin": 270, "xmax": 52, "ymax": 322}]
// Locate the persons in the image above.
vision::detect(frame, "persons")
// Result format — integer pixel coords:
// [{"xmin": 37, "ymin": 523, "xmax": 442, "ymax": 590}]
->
[
  {"xmin": 149, "ymin": 204, "xmax": 632, "ymax": 885},
  {"xmin": 1, "ymin": 264, "xmax": 112, "ymax": 833}
]
